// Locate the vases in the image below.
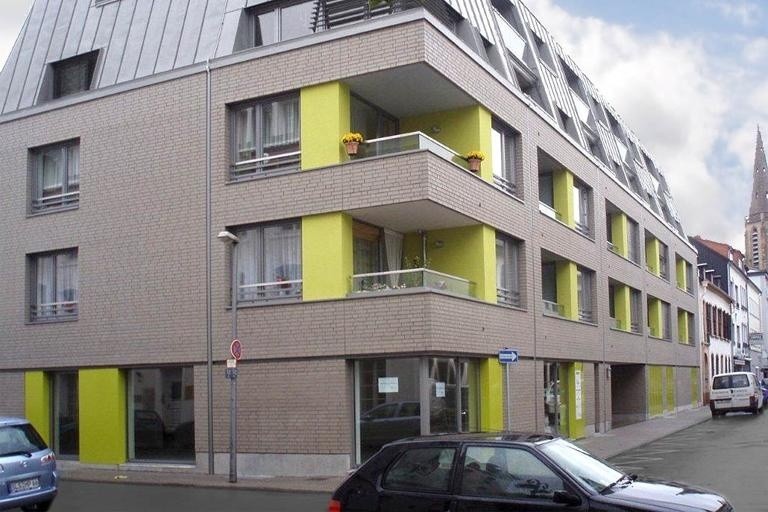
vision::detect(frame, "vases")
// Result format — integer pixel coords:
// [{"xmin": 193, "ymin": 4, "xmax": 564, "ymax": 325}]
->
[
  {"xmin": 345, "ymin": 142, "xmax": 359, "ymax": 154},
  {"xmin": 468, "ymin": 159, "xmax": 480, "ymax": 171}
]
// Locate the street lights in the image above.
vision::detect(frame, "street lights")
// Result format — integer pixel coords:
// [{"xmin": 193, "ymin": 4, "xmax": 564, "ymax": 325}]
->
[{"xmin": 214, "ymin": 230, "xmax": 242, "ymax": 483}]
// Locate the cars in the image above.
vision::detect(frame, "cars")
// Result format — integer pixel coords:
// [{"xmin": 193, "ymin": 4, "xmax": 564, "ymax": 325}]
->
[
  {"xmin": 166, "ymin": 419, "xmax": 194, "ymax": 458},
  {"xmin": 324, "ymin": 427, "xmax": 738, "ymax": 512},
  {"xmin": 359, "ymin": 400, "xmax": 449, "ymax": 449},
  {"xmin": 757, "ymin": 378, "xmax": 768, "ymax": 405},
  {"xmin": 130, "ymin": 406, "xmax": 169, "ymax": 452}
]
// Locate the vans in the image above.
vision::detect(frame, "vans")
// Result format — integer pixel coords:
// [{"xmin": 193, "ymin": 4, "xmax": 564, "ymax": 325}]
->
[
  {"xmin": 543, "ymin": 379, "xmax": 560, "ymax": 425},
  {"xmin": 0, "ymin": 412, "xmax": 58, "ymax": 512},
  {"xmin": 706, "ymin": 372, "xmax": 764, "ymax": 420}
]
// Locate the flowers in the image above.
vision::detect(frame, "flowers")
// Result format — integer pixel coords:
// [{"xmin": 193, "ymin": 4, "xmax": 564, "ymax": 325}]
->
[
  {"xmin": 467, "ymin": 150, "xmax": 485, "ymax": 160},
  {"xmin": 342, "ymin": 132, "xmax": 363, "ymax": 142}
]
